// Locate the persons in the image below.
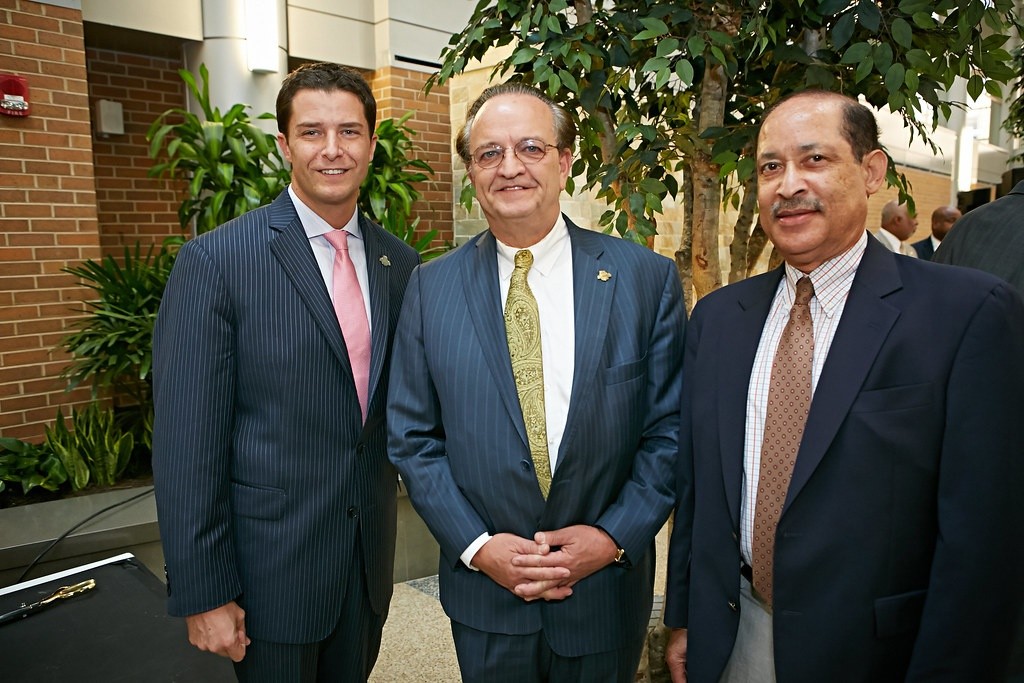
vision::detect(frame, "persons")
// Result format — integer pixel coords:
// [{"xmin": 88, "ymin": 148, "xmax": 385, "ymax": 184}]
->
[
  {"xmin": 874, "ymin": 199, "xmax": 917, "ymax": 259},
  {"xmin": 152, "ymin": 64, "xmax": 421, "ymax": 683},
  {"xmin": 911, "ymin": 206, "xmax": 962, "ymax": 261},
  {"xmin": 385, "ymin": 85, "xmax": 689, "ymax": 683},
  {"xmin": 929, "ymin": 180, "xmax": 1024, "ymax": 293},
  {"xmin": 668, "ymin": 91, "xmax": 1024, "ymax": 683}
]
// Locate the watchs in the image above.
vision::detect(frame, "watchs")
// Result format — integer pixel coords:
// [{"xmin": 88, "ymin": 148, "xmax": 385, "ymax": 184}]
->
[{"xmin": 614, "ymin": 549, "xmax": 629, "ymax": 564}]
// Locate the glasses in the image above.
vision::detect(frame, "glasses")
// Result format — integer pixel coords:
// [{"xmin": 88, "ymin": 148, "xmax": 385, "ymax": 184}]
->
[{"xmin": 469, "ymin": 138, "xmax": 558, "ymax": 169}]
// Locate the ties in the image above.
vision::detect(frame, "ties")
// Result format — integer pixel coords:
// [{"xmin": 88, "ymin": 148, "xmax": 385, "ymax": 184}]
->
[
  {"xmin": 900, "ymin": 243, "xmax": 904, "ymax": 253},
  {"xmin": 323, "ymin": 229, "xmax": 372, "ymax": 426},
  {"xmin": 753, "ymin": 276, "xmax": 815, "ymax": 607},
  {"xmin": 504, "ymin": 249, "xmax": 553, "ymax": 501}
]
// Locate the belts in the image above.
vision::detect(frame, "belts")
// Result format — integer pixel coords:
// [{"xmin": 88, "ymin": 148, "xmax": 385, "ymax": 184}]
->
[{"xmin": 741, "ymin": 565, "xmax": 752, "ymax": 586}]
